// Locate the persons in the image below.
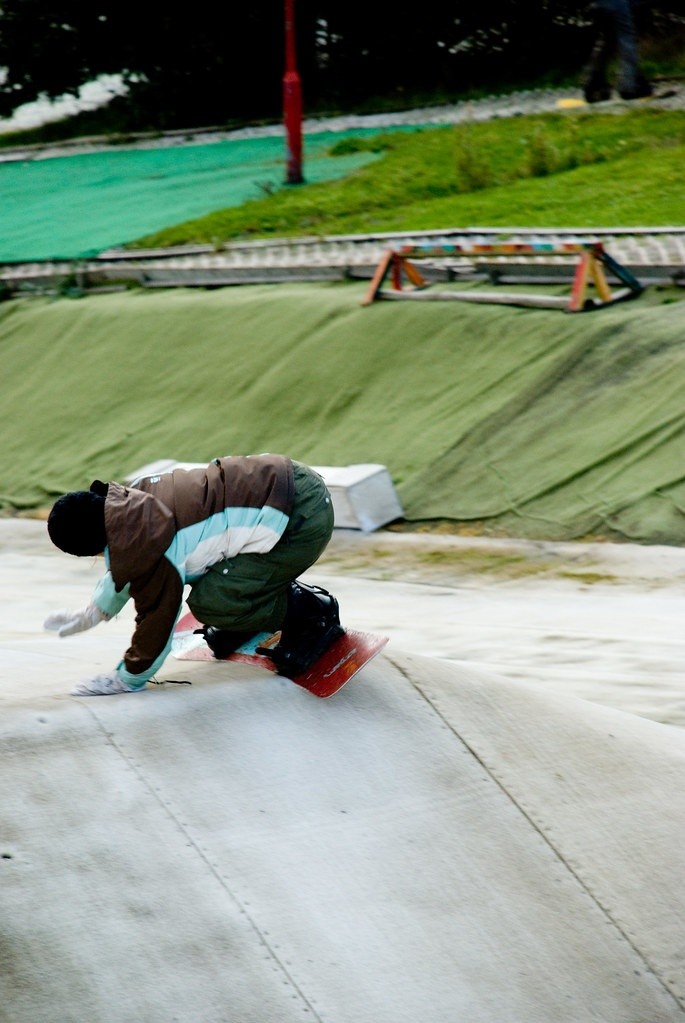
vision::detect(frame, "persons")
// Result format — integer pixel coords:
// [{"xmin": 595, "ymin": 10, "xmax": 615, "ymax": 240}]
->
[
  {"xmin": 44, "ymin": 453, "xmax": 345, "ymax": 696},
  {"xmin": 584, "ymin": 0, "xmax": 655, "ymax": 102}
]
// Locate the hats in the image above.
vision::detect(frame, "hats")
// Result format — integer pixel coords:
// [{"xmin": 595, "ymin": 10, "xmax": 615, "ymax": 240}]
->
[{"xmin": 47, "ymin": 490, "xmax": 107, "ymax": 557}]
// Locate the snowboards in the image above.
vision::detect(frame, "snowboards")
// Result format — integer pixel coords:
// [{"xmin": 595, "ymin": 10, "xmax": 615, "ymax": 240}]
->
[{"xmin": 168, "ymin": 608, "xmax": 391, "ymax": 701}]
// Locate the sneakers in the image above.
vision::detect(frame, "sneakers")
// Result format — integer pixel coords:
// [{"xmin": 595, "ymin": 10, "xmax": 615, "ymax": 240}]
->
[
  {"xmin": 193, "ymin": 624, "xmax": 260, "ymax": 650},
  {"xmin": 255, "ymin": 580, "xmax": 345, "ymax": 678}
]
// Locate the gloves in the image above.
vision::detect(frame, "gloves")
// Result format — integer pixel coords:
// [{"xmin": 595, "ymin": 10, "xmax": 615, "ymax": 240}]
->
[
  {"xmin": 70, "ymin": 673, "xmax": 145, "ymax": 696},
  {"xmin": 43, "ymin": 605, "xmax": 101, "ymax": 638}
]
